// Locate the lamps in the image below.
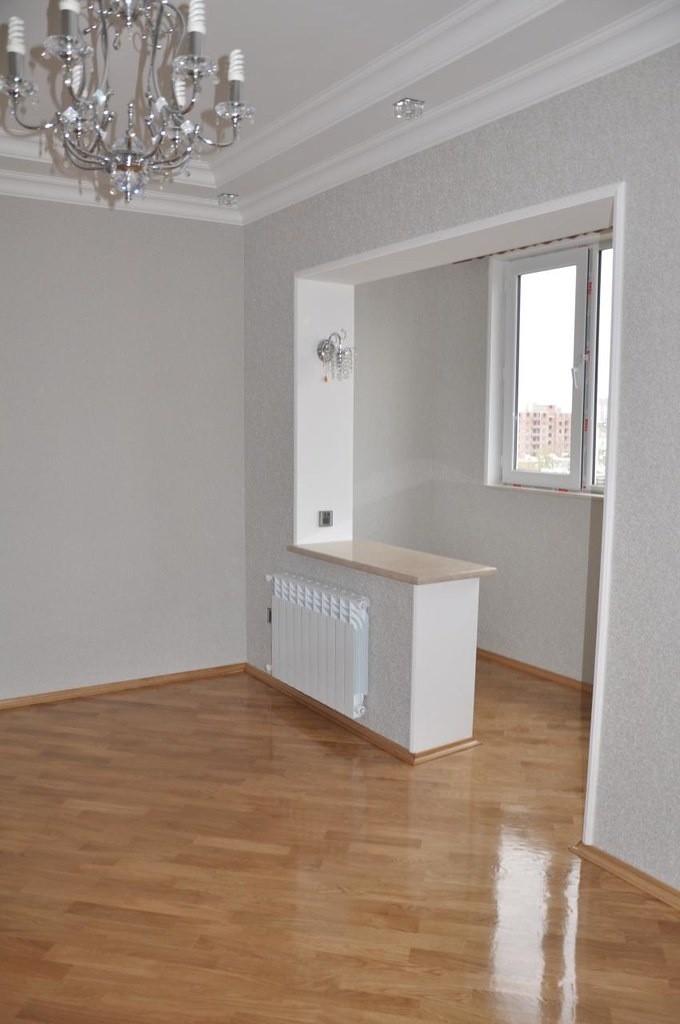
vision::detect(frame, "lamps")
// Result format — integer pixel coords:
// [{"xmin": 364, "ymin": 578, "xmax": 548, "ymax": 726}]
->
[
  {"xmin": 318, "ymin": 332, "xmax": 352, "ymax": 382},
  {"xmin": 0, "ymin": 0, "xmax": 255, "ymax": 203}
]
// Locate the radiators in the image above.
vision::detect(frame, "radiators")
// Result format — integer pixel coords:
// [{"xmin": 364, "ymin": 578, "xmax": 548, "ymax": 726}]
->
[{"xmin": 261, "ymin": 573, "xmax": 370, "ymax": 721}]
